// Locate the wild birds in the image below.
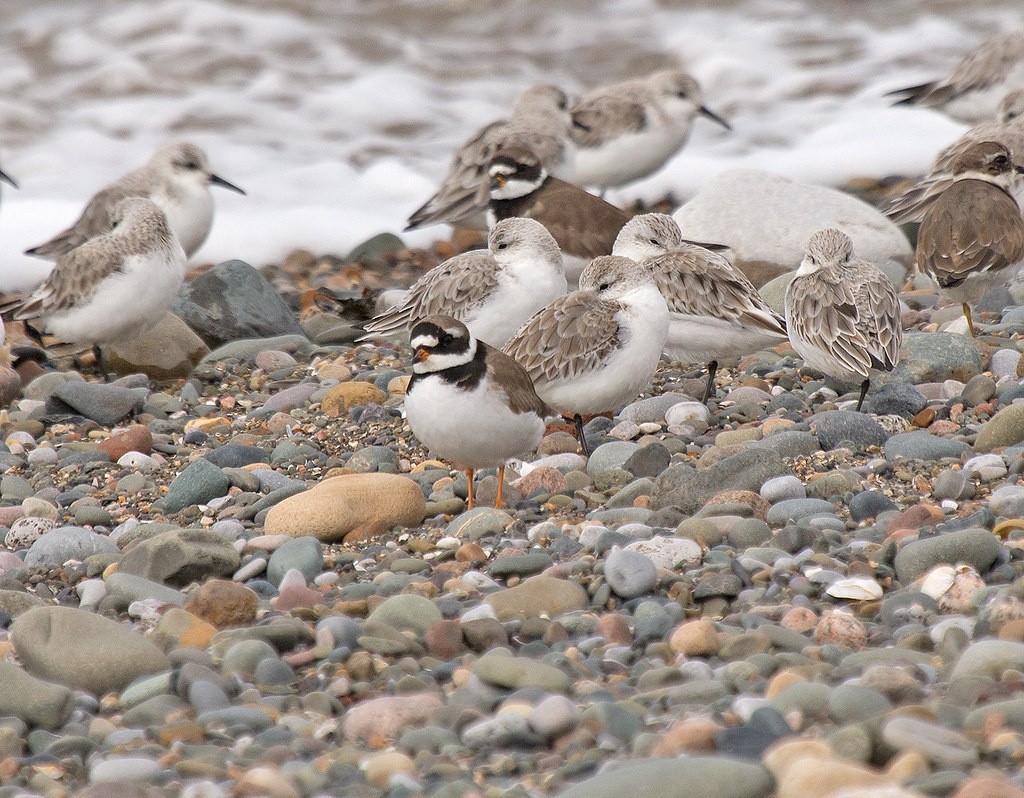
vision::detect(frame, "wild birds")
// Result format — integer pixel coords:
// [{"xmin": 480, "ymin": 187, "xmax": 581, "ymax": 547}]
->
[{"xmin": 1, "ymin": 33, "xmax": 1024, "ymax": 516}]
